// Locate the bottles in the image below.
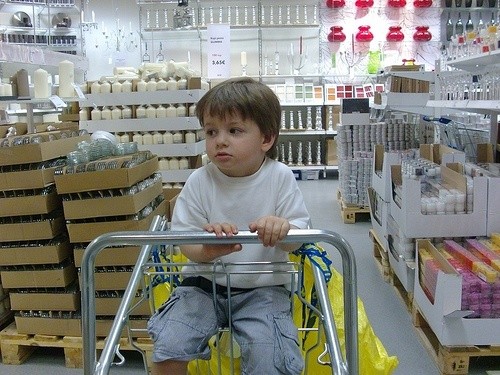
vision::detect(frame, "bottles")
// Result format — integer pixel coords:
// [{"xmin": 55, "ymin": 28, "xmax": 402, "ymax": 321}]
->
[{"xmin": 440, "ymin": 0, "xmax": 500, "ymax": 41}]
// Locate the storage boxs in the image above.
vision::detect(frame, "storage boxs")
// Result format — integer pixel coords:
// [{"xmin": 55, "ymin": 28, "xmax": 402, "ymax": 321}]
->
[
  {"xmin": 325, "ymin": 106, "xmax": 342, "ymax": 130},
  {"xmin": 0, "ymin": 121, "xmax": 182, "ymax": 337},
  {"xmin": 367, "ymin": 143, "xmax": 500, "ymax": 348},
  {"xmin": 325, "ymin": 137, "xmax": 338, "ymax": 165}
]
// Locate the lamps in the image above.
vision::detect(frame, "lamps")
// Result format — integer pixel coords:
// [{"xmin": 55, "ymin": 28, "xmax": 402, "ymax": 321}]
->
[{"xmin": 81, "ymin": 8, "xmax": 144, "ymax": 54}]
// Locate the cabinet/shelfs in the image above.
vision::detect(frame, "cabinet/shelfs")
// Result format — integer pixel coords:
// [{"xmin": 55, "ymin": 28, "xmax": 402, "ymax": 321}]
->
[
  {"xmin": 273, "ymin": 103, "xmax": 341, "ymax": 172},
  {"xmin": 139, "ymin": 0, "xmax": 321, "ymax": 85},
  {"xmin": 0, "ymin": 0, "xmax": 84, "ymax": 57},
  {"xmin": 79, "ymin": 77, "xmax": 210, "ymax": 189},
  {"xmin": 369, "ymin": 48, "xmax": 500, "ymax": 162}
]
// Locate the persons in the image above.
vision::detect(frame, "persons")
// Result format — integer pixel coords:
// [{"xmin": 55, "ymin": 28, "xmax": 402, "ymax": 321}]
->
[{"xmin": 147, "ymin": 78, "xmax": 312, "ymax": 375}]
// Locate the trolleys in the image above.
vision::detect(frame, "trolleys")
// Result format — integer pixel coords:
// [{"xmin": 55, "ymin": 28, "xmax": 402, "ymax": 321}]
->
[{"xmin": 77, "ymin": 214, "xmax": 362, "ymax": 375}]
